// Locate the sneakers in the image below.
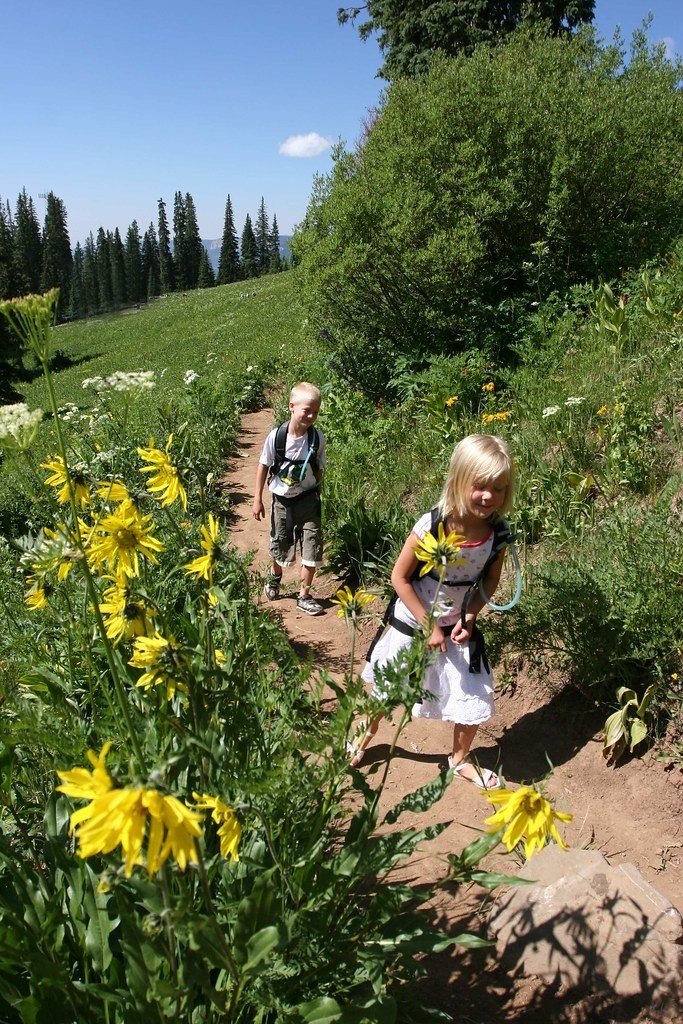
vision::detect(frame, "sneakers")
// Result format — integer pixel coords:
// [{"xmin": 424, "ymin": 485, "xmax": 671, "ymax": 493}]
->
[
  {"xmin": 296, "ymin": 594, "xmax": 323, "ymax": 615},
  {"xmin": 264, "ymin": 560, "xmax": 282, "ymax": 601}
]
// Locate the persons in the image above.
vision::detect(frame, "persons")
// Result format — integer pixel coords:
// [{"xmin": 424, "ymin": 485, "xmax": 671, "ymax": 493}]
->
[
  {"xmin": 342, "ymin": 435, "xmax": 515, "ymax": 790},
  {"xmin": 252, "ymin": 382, "xmax": 327, "ymax": 615}
]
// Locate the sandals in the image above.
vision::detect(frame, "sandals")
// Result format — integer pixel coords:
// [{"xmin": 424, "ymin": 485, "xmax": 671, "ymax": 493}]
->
[
  {"xmin": 448, "ymin": 755, "xmax": 502, "ymax": 791},
  {"xmin": 343, "ymin": 720, "xmax": 378, "ymax": 766}
]
